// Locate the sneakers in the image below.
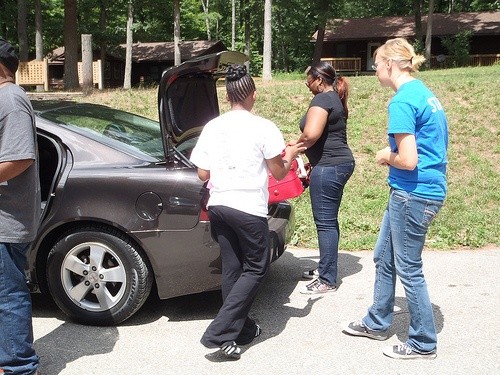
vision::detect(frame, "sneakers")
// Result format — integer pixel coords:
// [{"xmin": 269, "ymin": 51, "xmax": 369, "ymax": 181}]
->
[
  {"xmin": 383, "ymin": 343, "xmax": 437, "ymax": 359},
  {"xmin": 255, "ymin": 325, "xmax": 261, "ymax": 337},
  {"xmin": 299, "ymin": 269, "xmax": 336, "ymax": 294},
  {"xmin": 218, "ymin": 340, "xmax": 242, "ymax": 359},
  {"xmin": 342, "ymin": 318, "xmax": 388, "ymax": 340}
]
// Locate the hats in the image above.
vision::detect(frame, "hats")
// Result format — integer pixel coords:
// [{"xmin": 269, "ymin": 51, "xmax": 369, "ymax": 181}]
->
[{"xmin": 0, "ymin": 38, "xmax": 19, "ymax": 73}]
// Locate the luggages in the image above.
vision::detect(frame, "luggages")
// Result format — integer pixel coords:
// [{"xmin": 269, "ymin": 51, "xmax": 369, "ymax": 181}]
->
[{"xmin": 266, "ymin": 151, "xmax": 309, "ymax": 206}]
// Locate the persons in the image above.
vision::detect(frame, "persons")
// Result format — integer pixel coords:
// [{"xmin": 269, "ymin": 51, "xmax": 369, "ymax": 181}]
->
[
  {"xmin": 0, "ymin": 38, "xmax": 41, "ymax": 375},
  {"xmin": 188, "ymin": 66, "xmax": 307, "ymax": 358},
  {"xmin": 288, "ymin": 63, "xmax": 356, "ymax": 295},
  {"xmin": 342, "ymin": 38, "xmax": 448, "ymax": 359}
]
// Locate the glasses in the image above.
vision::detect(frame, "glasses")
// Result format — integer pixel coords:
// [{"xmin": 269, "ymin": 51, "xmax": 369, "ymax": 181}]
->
[
  {"xmin": 371, "ymin": 59, "xmax": 383, "ymax": 71},
  {"xmin": 305, "ymin": 78, "xmax": 316, "ymax": 87}
]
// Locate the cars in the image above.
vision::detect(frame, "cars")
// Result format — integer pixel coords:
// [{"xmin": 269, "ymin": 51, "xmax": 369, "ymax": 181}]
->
[{"xmin": 26, "ymin": 48, "xmax": 294, "ymax": 326}]
